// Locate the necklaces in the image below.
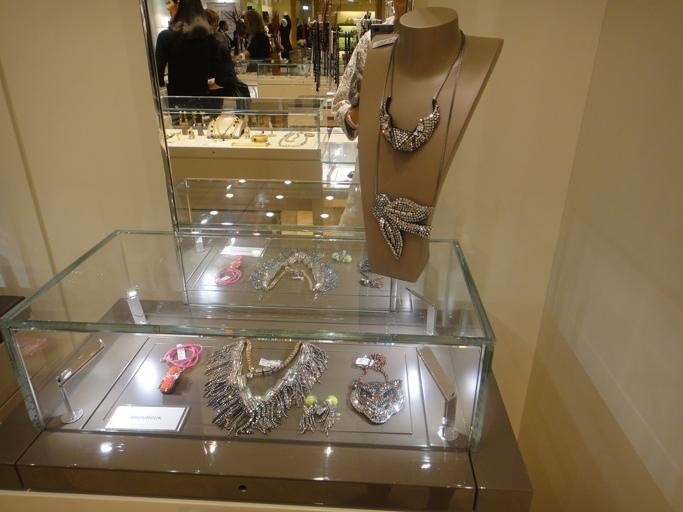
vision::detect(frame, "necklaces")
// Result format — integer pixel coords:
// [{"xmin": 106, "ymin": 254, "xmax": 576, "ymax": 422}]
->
[
  {"xmin": 248, "ymin": 246, "xmax": 341, "ymax": 299},
  {"xmin": 198, "ymin": 338, "xmax": 328, "ymax": 439},
  {"xmin": 277, "ymin": 132, "xmax": 307, "ymax": 148},
  {"xmin": 363, "ymin": 27, "xmax": 467, "ymax": 260},
  {"xmin": 348, "ymin": 351, "xmax": 405, "ymax": 426},
  {"xmin": 209, "ymin": 114, "xmax": 239, "ymax": 142},
  {"xmin": 212, "ymin": 255, "xmax": 243, "ymax": 288},
  {"xmin": 309, "ymin": 22, "xmax": 359, "ymax": 93},
  {"xmin": 157, "ymin": 342, "xmax": 203, "ymax": 394},
  {"xmin": 354, "ymin": 256, "xmax": 388, "ymax": 289}
]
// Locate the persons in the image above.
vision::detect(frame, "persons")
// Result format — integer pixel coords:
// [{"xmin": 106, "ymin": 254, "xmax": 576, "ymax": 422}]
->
[
  {"xmin": 278, "ymin": 13, "xmax": 293, "ymax": 53},
  {"xmin": 214, "ymin": 20, "xmax": 233, "ymax": 50},
  {"xmin": 164, "ymin": 1, "xmax": 184, "ymax": 33},
  {"xmin": 231, "ymin": 9, "xmax": 272, "ymax": 72},
  {"xmin": 198, "ymin": 8, "xmax": 233, "ymax": 85},
  {"xmin": 330, "ymin": 17, "xmax": 399, "ymax": 143},
  {"xmin": 356, "ymin": 6, "xmax": 504, "ymax": 284},
  {"xmin": 153, "ymin": 1, "xmax": 234, "ymax": 125}
]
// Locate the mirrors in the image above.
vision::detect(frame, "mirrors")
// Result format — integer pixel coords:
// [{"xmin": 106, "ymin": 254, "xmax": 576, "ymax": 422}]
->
[{"xmin": 135, "ymin": 0, "xmax": 416, "ymax": 318}]
